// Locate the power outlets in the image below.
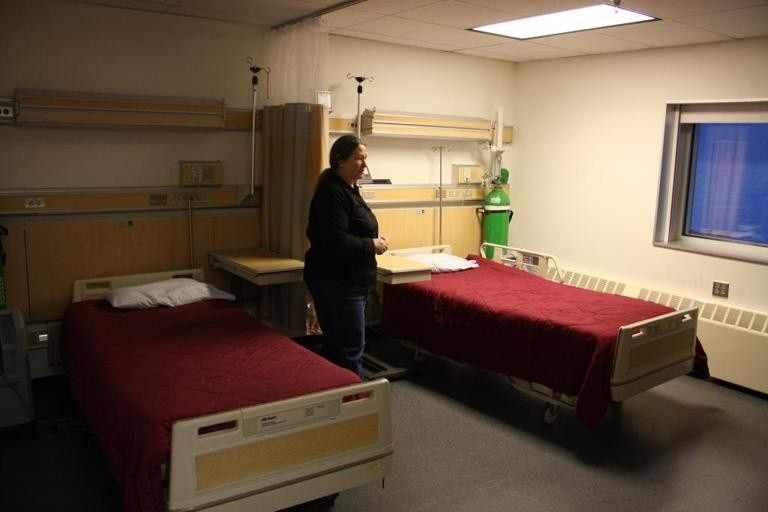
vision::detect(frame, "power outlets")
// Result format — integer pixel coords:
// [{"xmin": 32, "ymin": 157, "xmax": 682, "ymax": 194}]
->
[{"xmin": 711, "ymin": 281, "xmax": 728, "ymax": 300}]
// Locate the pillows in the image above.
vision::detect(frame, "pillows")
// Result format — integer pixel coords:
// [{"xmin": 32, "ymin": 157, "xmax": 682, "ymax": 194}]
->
[
  {"xmin": 102, "ymin": 278, "xmax": 236, "ymax": 311},
  {"xmin": 385, "ymin": 250, "xmax": 480, "ymax": 274}
]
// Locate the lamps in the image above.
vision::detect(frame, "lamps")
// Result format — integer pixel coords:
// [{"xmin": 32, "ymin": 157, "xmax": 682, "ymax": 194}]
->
[{"xmin": 465, "ymin": 2, "xmax": 664, "ymax": 42}]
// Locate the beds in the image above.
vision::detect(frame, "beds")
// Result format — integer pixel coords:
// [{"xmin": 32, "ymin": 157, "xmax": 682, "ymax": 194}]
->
[
  {"xmin": 59, "ymin": 267, "xmax": 393, "ymax": 512},
  {"xmin": 367, "ymin": 248, "xmax": 699, "ymax": 442}
]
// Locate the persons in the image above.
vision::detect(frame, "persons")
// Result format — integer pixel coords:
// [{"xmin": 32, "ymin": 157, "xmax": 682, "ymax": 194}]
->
[{"xmin": 301, "ymin": 135, "xmax": 389, "ymax": 378}]
